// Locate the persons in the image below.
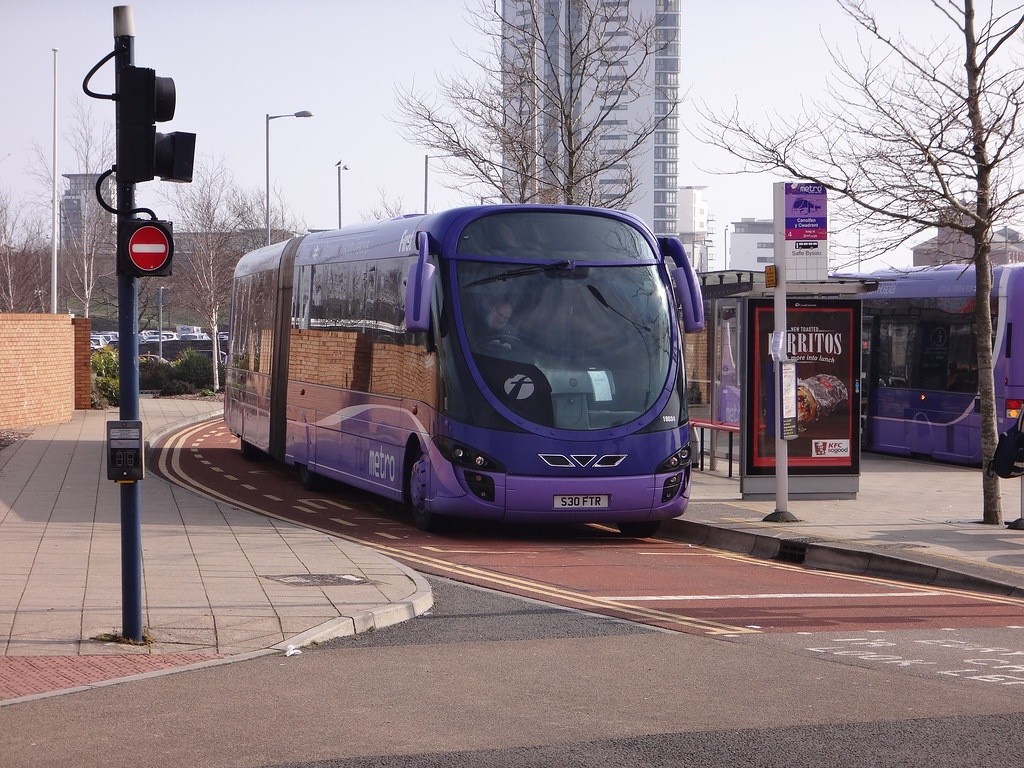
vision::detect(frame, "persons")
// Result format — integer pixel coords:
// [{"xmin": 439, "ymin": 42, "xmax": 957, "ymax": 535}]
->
[{"xmin": 471, "ymin": 299, "xmax": 518, "ymax": 351}]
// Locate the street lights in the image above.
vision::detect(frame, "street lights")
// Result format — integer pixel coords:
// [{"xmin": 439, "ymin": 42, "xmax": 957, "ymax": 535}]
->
[
  {"xmin": 424, "ymin": 151, "xmax": 469, "ymax": 215},
  {"xmin": 266, "ymin": 111, "xmax": 313, "ymax": 246}
]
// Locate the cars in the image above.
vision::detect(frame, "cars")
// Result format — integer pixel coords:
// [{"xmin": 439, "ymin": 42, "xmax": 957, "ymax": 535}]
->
[{"xmin": 90, "ymin": 325, "xmax": 229, "ymax": 350}]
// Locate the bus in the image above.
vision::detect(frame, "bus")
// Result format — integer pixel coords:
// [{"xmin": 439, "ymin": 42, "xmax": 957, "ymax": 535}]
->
[
  {"xmin": 716, "ymin": 263, "xmax": 1024, "ymax": 470},
  {"xmin": 225, "ymin": 203, "xmax": 705, "ymax": 539}
]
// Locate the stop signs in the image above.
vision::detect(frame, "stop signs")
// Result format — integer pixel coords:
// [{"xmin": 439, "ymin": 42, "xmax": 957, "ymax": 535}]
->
[{"xmin": 116, "ymin": 219, "xmax": 174, "ymax": 277}]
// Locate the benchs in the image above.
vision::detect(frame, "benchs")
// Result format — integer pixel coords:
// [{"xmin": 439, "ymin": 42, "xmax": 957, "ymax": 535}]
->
[{"xmin": 687, "ymin": 419, "xmax": 740, "ymax": 477}]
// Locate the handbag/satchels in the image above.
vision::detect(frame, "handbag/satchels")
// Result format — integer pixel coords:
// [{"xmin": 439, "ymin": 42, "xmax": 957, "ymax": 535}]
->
[{"xmin": 987, "ymin": 405, "xmax": 1024, "ymax": 479}]
